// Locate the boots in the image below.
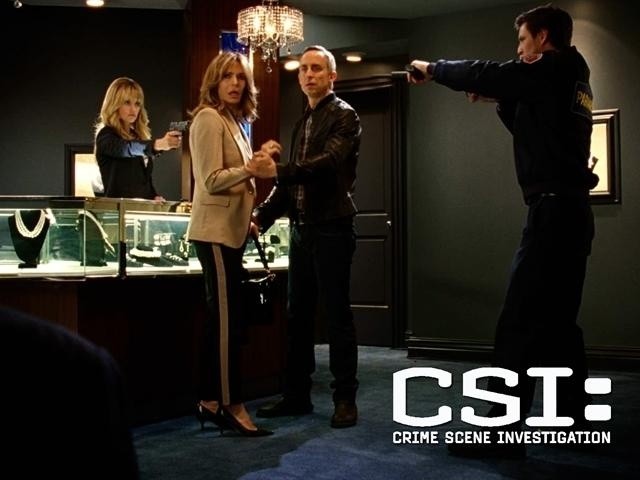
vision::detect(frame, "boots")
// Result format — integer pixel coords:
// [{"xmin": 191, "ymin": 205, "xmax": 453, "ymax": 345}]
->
[{"xmin": 448, "ymin": 403, "xmax": 526, "ymax": 462}]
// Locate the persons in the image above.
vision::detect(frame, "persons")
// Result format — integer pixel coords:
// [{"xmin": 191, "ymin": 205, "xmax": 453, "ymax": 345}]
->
[
  {"xmin": 186, "ymin": 51, "xmax": 284, "ymax": 437},
  {"xmin": 251, "ymin": 44, "xmax": 363, "ymax": 429},
  {"xmin": 96, "ymin": 76, "xmax": 184, "ymax": 203},
  {"xmin": 405, "ymin": 2, "xmax": 600, "ymax": 459}
]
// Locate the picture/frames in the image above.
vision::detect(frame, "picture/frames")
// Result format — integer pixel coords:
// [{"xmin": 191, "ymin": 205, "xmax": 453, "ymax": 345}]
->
[{"xmin": 586, "ymin": 108, "xmax": 623, "ymax": 205}]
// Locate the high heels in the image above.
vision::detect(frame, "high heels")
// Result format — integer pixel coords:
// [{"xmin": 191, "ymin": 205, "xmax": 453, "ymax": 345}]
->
[{"xmin": 197, "ymin": 404, "xmax": 274, "ymax": 437}]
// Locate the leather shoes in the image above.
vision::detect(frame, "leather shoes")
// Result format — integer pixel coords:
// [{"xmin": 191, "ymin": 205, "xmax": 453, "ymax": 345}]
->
[
  {"xmin": 331, "ymin": 400, "xmax": 357, "ymax": 427},
  {"xmin": 256, "ymin": 395, "xmax": 314, "ymax": 418}
]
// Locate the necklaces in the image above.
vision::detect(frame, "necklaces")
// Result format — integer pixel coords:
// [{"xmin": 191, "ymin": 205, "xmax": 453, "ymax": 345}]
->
[
  {"xmin": 13, "ymin": 209, "xmax": 46, "ymax": 239},
  {"xmin": 79, "ymin": 210, "xmax": 109, "ymax": 239},
  {"xmin": 130, "ymin": 247, "xmax": 161, "ymax": 258}
]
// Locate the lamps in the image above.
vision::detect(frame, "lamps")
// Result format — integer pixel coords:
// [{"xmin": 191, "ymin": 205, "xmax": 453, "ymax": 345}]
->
[{"xmin": 236, "ymin": 1, "xmax": 306, "ymax": 77}]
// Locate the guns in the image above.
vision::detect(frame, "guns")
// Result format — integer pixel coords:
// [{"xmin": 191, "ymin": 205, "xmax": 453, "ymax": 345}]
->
[
  {"xmin": 168, "ymin": 121, "xmax": 190, "ymax": 151},
  {"xmin": 372, "ymin": 65, "xmax": 425, "ymax": 82}
]
